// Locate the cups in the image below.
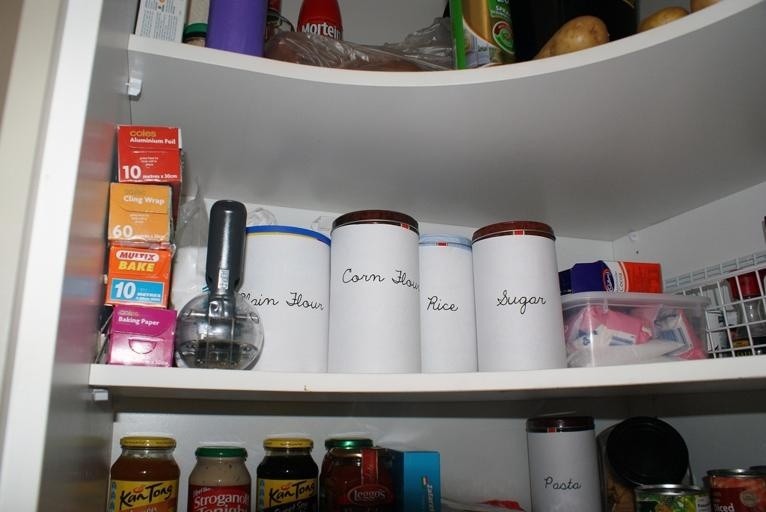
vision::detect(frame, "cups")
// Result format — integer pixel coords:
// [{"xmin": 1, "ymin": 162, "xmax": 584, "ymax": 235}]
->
[{"xmin": 204, "ymin": 0, "xmax": 268, "ymax": 58}]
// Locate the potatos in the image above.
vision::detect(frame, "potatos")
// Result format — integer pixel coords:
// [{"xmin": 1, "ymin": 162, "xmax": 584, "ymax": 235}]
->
[
  {"xmin": 691, "ymin": 0, "xmax": 719, "ymax": 12},
  {"xmin": 639, "ymin": 7, "xmax": 688, "ymax": 32},
  {"xmin": 531, "ymin": 15, "xmax": 609, "ymax": 59}
]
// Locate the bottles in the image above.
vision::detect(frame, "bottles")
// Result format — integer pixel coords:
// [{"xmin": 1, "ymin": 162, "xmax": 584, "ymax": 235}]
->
[
  {"xmin": 524, "ymin": 414, "xmax": 599, "ymax": 512},
  {"xmin": 472, "ymin": 221, "xmax": 567, "ymax": 373},
  {"xmin": 418, "ymin": 232, "xmax": 477, "ymax": 372},
  {"xmin": 730, "ymin": 270, "xmax": 765, "ymax": 344},
  {"xmin": 233, "ymin": 223, "xmax": 330, "ymax": 373},
  {"xmin": 594, "ymin": 416, "xmax": 694, "ymax": 510},
  {"xmin": 330, "ymin": 210, "xmax": 420, "ymax": 372},
  {"xmin": 296, "ymin": 1, "xmax": 342, "ymax": 42}
]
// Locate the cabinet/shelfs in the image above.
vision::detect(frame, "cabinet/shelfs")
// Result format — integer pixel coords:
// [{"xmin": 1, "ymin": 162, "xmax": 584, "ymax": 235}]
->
[{"xmin": 1, "ymin": 1, "xmax": 766, "ymax": 510}]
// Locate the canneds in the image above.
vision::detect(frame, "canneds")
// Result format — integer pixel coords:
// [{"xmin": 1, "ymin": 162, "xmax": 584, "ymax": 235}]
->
[
  {"xmin": 449, "ymin": 0, "xmax": 515, "ymax": 69},
  {"xmin": 256, "ymin": 439, "xmax": 318, "ymax": 511},
  {"xmin": 634, "ymin": 484, "xmax": 699, "ymax": 512},
  {"xmin": 701, "ymin": 465, "xmax": 766, "ymax": 511},
  {"xmin": 107, "ymin": 435, "xmax": 179, "ymax": 511},
  {"xmin": 187, "ymin": 445, "xmax": 251, "ymax": 511},
  {"xmin": 321, "ymin": 437, "xmax": 395, "ymax": 512},
  {"xmin": 696, "ymin": 490, "xmax": 712, "ymax": 512}
]
